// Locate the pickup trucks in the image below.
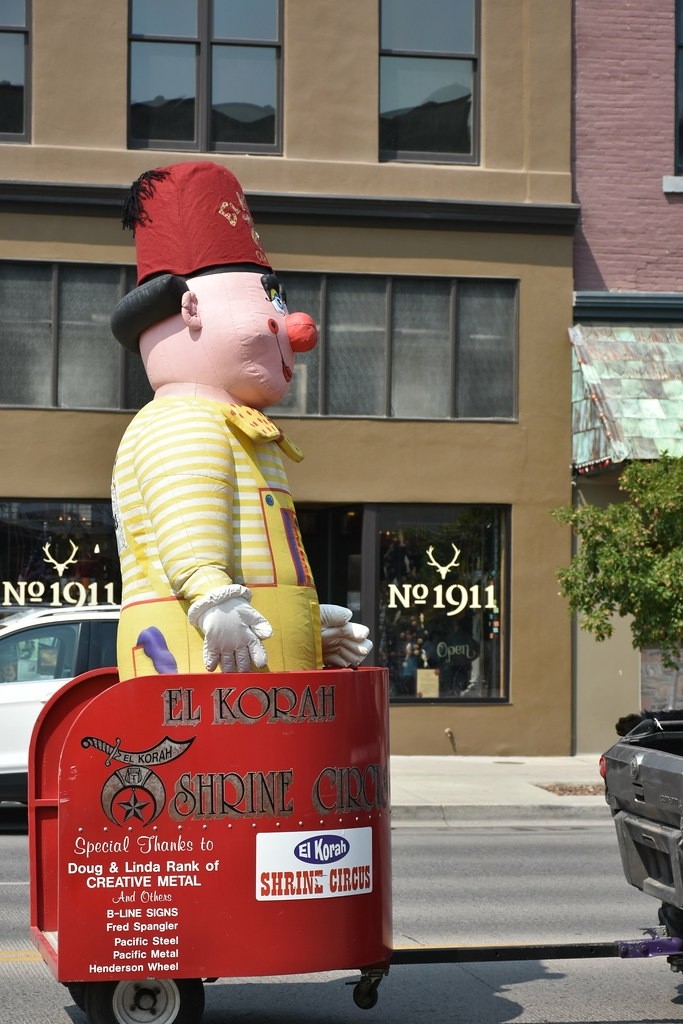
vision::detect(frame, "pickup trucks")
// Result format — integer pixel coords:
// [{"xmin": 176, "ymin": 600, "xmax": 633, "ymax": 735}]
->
[{"xmin": 599, "ymin": 716, "xmax": 682, "ymax": 971}]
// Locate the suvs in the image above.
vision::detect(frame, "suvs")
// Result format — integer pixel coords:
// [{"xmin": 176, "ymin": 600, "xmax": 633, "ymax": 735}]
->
[{"xmin": 1, "ymin": 604, "xmax": 123, "ymax": 807}]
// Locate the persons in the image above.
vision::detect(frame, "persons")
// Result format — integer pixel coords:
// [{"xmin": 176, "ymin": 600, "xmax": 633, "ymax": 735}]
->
[{"xmin": 115, "ymin": 165, "xmax": 374, "ymax": 683}]
